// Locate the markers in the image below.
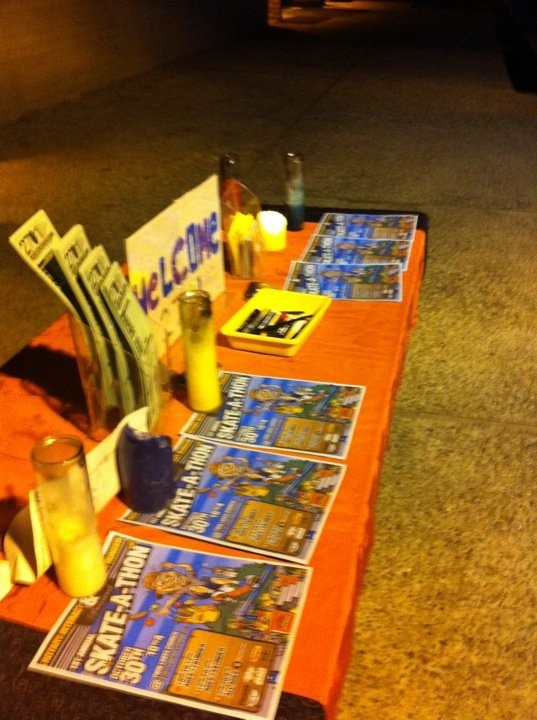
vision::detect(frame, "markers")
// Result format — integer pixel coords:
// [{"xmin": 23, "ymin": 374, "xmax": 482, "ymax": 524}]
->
[{"xmin": 236, "ymin": 308, "xmax": 316, "ymax": 340}]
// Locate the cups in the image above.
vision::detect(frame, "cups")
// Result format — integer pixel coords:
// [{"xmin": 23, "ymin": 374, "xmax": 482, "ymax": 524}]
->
[
  {"xmin": 29, "ymin": 434, "xmax": 107, "ymax": 597},
  {"xmin": 219, "ymin": 147, "xmax": 304, "ymax": 280},
  {"xmin": 179, "ymin": 290, "xmax": 221, "ymax": 412}
]
